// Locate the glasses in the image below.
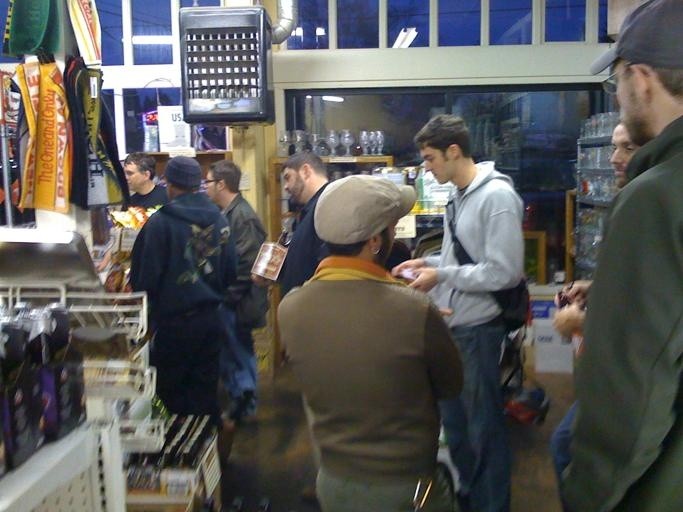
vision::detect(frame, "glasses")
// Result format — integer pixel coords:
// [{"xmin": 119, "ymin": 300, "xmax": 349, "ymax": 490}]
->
[
  {"xmin": 599, "ymin": 61, "xmax": 638, "ymax": 97},
  {"xmin": 202, "ymin": 178, "xmax": 222, "ymax": 188}
]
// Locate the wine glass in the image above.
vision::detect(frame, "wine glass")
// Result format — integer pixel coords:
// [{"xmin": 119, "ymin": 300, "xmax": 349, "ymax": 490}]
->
[
  {"xmin": 328, "ymin": 169, "xmax": 382, "ymax": 180},
  {"xmin": 279, "ymin": 129, "xmax": 386, "ymax": 157}
]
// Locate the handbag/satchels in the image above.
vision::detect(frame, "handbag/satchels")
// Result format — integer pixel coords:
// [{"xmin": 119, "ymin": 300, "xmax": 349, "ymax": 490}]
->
[{"xmin": 498, "ymin": 274, "xmax": 530, "ymax": 333}]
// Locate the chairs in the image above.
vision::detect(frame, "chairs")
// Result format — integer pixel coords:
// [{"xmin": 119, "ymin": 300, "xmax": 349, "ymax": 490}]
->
[{"xmin": 523, "ymin": 230, "xmax": 546, "ymax": 284}]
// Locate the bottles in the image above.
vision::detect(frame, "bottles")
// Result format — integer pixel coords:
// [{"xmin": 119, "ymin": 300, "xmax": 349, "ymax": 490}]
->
[{"xmin": 276, "ymin": 228, "xmax": 289, "ymax": 245}]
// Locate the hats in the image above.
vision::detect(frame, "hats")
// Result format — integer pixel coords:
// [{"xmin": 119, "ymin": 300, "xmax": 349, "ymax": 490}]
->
[
  {"xmin": 158, "ymin": 155, "xmax": 201, "ymax": 189},
  {"xmin": 312, "ymin": 176, "xmax": 417, "ymax": 247},
  {"xmin": 586, "ymin": 1, "xmax": 682, "ymax": 77}
]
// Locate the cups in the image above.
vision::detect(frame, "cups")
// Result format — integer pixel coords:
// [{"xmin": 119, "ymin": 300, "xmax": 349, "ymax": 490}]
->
[{"xmin": 573, "ymin": 112, "xmax": 619, "ymax": 287}]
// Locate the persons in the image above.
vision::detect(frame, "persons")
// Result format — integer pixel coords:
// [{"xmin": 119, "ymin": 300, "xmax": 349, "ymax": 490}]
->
[
  {"xmin": 560, "ymin": 0, "xmax": 683, "ymax": 512},
  {"xmin": 130, "ymin": 155, "xmax": 239, "ymax": 433},
  {"xmin": 204, "ymin": 160, "xmax": 269, "ymax": 418},
  {"xmin": 122, "ymin": 153, "xmax": 170, "ymax": 207},
  {"xmin": 552, "ymin": 122, "xmax": 642, "ymax": 509}
]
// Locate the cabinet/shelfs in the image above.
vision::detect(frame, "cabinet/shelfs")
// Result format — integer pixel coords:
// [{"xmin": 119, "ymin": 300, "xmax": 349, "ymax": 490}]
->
[
  {"xmin": 268, "ymin": 155, "xmax": 392, "ymax": 373},
  {"xmin": 0, "ymin": 397, "xmax": 128, "ymax": 512},
  {"xmin": 573, "ymin": 135, "xmax": 621, "ymax": 280}
]
